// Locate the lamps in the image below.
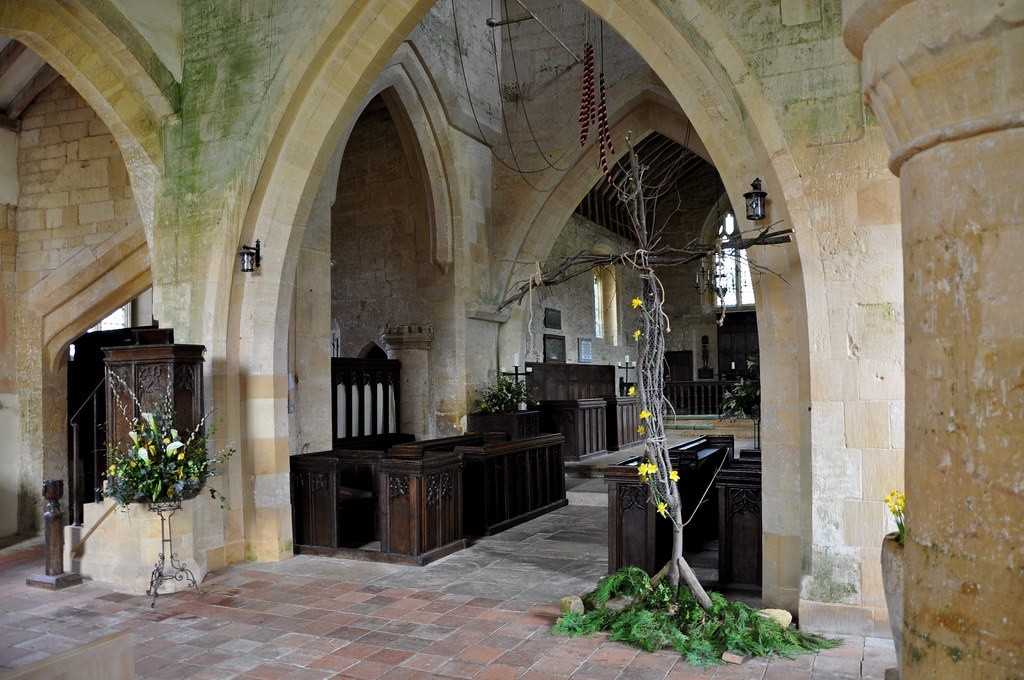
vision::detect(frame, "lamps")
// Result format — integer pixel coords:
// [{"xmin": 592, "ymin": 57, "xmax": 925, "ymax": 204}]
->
[
  {"xmin": 743, "ymin": 177, "xmax": 767, "ymax": 220},
  {"xmin": 239, "ymin": 238, "xmax": 260, "ymax": 272}
]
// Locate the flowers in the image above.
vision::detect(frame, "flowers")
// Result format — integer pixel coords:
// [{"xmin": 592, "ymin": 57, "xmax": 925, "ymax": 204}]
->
[
  {"xmin": 93, "ymin": 366, "xmax": 238, "ymax": 528},
  {"xmin": 883, "ymin": 489, "xmax": 905, "ymax": 546}
]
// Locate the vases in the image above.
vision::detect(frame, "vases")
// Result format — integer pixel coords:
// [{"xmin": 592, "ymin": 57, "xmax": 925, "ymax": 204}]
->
[
  {"xmin": 880, "ymin": 531, "xmax": 904, "ymax": 680},
  {"xmin": 119, "ymin": 481, "xmax": 204, "ymax": 502}
]
